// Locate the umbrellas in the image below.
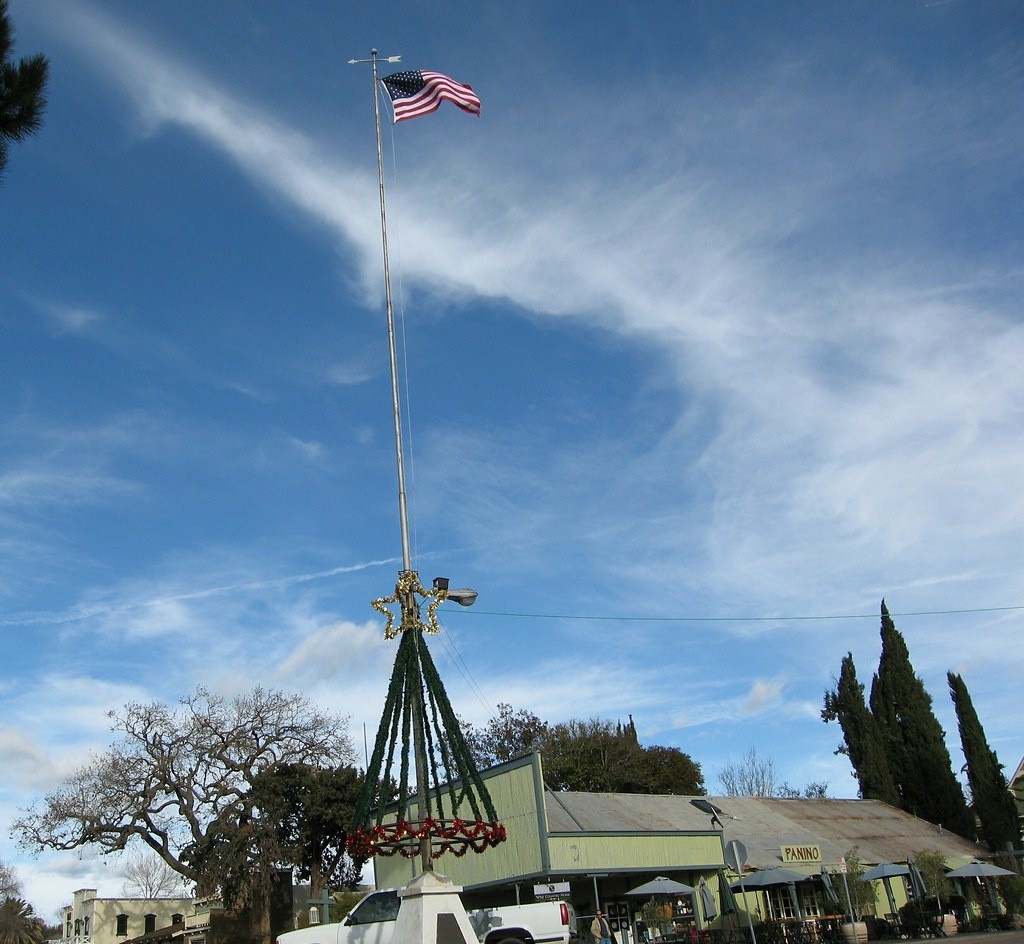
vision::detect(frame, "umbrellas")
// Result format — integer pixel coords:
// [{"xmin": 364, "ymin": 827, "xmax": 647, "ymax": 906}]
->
[
  {"xmin": 857, "ymin": 862, "xmax": 923, "ymax": 926},
  {"xmin": 821, "ymin": 863, "xmax": 840, "ymax": 917},
  {"xmin": 730, "ymin": 865, "xmax": 811, "ymax": 924},
  {"xmin": 717, "ymin": 867, "xmax": 741, "ymax": 932},
  {"xmin": 945, "ymin": 860, "xmax": 1018, "ymax": 915},
  {"xmin": 906, "ymin": 856, "xmax": 930, "ymax": 938},
  {"xmin": 625, "ymin": 876, "xmax": 698, "ymax": 933},
  {"xmin": 698, "ymin": 875, "xmax": 717, "ymax": 927}
]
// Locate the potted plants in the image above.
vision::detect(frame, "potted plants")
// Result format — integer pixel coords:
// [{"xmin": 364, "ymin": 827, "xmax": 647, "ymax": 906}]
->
[
  {"xmin": 831, "ymin": 845, "xmax": 879, "ymax": 944},
  {"xmin": 1001, "ymin": 875, "xmax": 1024, "ymax": 930},
  {"xmin": 913, "ymin": 847, "xmax": 958, "ymax": 936}
]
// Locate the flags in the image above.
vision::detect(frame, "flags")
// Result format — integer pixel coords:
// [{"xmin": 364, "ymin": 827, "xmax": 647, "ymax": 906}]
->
[{"xmin": 380, "ymin": 70, "xmax": 481, "ymax": 122}]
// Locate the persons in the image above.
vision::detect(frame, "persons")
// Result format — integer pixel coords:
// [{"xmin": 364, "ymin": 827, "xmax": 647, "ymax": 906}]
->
[{"xmin": 590, "ymin": 910, "xmax": 612, "ymax": 944}]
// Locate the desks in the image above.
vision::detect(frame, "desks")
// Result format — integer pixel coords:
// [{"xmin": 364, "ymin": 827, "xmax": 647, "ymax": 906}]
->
[{"xmin": 817, "ymin": 915, "xmax": 844, "ymax": 944}]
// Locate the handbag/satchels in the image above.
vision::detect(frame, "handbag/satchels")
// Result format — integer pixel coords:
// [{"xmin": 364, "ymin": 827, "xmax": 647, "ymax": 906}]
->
[{"xmin": 595, "ymin": 937, "xmax": 601, "ymax": 944}]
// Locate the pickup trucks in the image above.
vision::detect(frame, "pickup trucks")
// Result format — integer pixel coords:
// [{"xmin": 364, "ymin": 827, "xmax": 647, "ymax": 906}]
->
[{"xmin": 276, "ymin": 886, "xmax": 580, "ymax": 944}]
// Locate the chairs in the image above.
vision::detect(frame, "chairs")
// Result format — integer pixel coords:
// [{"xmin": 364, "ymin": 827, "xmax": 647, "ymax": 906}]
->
[
  {"xmin": 662, "ymin": 934, "xmax": 678, "ymax": 944},
  {"xmin": 982, "ymin": 904, "xmax": 1000, "ymax": 932},
  {"xmin": 709, "ymin": 929, "xmax": 727, "ymax": 944},
  {"xmin": 884, "ymin": 902, "xmax": 948, "ymax": 940}
]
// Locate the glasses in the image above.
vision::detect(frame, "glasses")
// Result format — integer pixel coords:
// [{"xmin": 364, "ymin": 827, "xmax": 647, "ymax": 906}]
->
[{"xmin": 597, "ymin": 914, "xmax": 602, "ymax": 915}]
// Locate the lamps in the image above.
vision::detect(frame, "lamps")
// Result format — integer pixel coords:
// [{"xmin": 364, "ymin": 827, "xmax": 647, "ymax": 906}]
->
[{"xmin": 307, "ymin": 907, "xmax": 320, "ymax": 925}]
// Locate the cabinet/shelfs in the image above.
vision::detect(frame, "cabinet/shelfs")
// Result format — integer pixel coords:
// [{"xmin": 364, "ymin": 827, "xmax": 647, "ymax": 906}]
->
[{"xmin": 633, "ymin": 915, "xmax": 695, "ymax": 944}]
